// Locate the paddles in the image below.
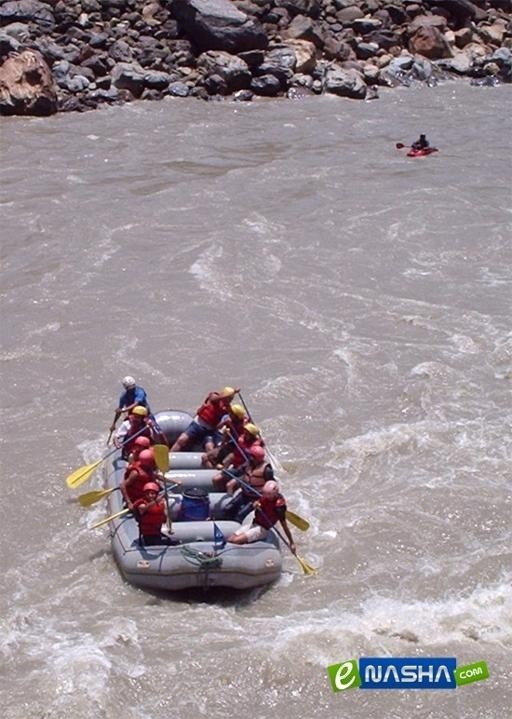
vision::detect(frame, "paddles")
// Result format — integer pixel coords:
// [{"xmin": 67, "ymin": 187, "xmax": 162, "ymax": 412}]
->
[
  {"xmin": 258, "ymin": 508, "xmax": 314, "ymax": 575},
  {"xmin": 79, "ymin": 485, "xmax": 122, "ymax": 508},
  {"xmin": 65, "ymin": 425, "xmax": 147, "ymax": 488},
  {"xmin": 238, "ymin": 392, "xmax": 282, "ymax": 471},
  {"xmin": 152, "ymin": 443, "xmax": 172, "ymax": 535},
  {"xmin": 89, "ymin": 481, "xmax": 178, "ymax": 531},
  {"xmin": 220, "ymin": 468, "xmax": 309, "ymax": 532}
]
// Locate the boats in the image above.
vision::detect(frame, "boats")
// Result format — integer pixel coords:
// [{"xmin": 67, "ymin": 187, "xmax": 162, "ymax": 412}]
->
[
  {"xmin": 406, "ymin": 148, "xmax": 438, "ymax": 157},
  {"xmin": 101, "ymin": 409, "xmax": 284, "ymax": 588}
]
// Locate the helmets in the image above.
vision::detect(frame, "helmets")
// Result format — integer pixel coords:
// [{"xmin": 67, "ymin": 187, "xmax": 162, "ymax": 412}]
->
[
  {"xmin": 142, "ymin": 482, "xmax": 160, "ymax": 493},
  {"xmin": 245, "ymin": 445, "xmax": 265, "ymax": 463},
  {"xmin": 138, "ymin": 449, "xmax": 155, "ymax": 465},
  {"xmin": 243, "ymin": 423, "xmax": 258, "ymax": 439},
  {"xmin": 223, "ymin": 387, "xmax": 235, "ymax": 400},
  {"xmin": 132, "ymin": 406, "xmax": 148, "ymax": 416},
  {"xmin": 134, "ymin": 436, "xmax": 151, "ymax": 447},
  {"xmin": 262, "ymin": 480, "xmax": 279, "ymax": 498},
  {"xmin": 121, "ymin": 376, "xmax": 136, "ymax": 390},
  {"xmin": 230, "ymin": 404, "xmax": 245, "ymax": 420}
]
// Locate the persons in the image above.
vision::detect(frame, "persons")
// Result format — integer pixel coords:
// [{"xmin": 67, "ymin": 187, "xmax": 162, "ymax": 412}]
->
[
  {"xmin": 225, "ymin": 480, "xmax": 297, "ymax": 555},
  {"xmin": 109, "ymin": 375, "xmax": 169, "ymax": 447},
  {"xmin": 112, "ymin": 405, "xmax": 162, "ymax": 463},
  {"xmin": 120, "ymin": 450, "xmax": 183, "ymax": 512},
  {"xmin": 225, "ymin": 443, "xmax": 277, "ymax": 525},
  {"xmin": 213, "ymin": 422, "xmax": 267, "ymax": 495},
  {"xmin": 131, "ymin": 480, "xmax": 176, "ymax": 547},
  {"xmin": 126, "ymin": 436, "xmax": 157, "ymax": 466},
  {"xmin": 199, "ymin": 404, "xmax": 249, "ymax": 470},
  {"xmin": 166, "ymin": 386, "xmax": 249, "ymax": 453},
  {"xmin": 409, "ymin": 133, "xmax": 431, "ymax": 152}
]
